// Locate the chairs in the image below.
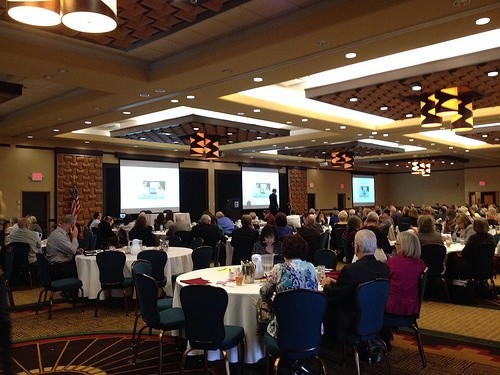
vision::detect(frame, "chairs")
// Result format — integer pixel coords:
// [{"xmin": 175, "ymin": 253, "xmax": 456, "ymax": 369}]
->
[{"xmin": 0, "ymin": 228, "xmax": 500, "ymax": 375}]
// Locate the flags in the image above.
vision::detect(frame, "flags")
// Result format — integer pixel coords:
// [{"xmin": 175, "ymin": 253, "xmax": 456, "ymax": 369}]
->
[{"xmin": 69, "ymin": 178, "xmax": 81, "ymax": 241}]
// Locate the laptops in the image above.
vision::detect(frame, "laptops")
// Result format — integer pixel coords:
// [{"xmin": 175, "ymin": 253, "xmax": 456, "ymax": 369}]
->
[{"xmin": 120, "ymin": 213, "xmax": 126, "ymax": 219}]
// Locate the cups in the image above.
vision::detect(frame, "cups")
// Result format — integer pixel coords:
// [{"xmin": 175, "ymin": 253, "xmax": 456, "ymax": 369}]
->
[
  {"xmin": 243, "ymin": 263, "xmax": 256, "ymax": 284},
  {"xmin": 317, "ymin": 265, "xmax": 325, "ymax": 283},
  {"xmin": 160, "ymin": 241, "xmax": 169, "ymax": 252},
  {"xmin": 461, "ymin": 240, "xmax": 464, "ymax": 245},
  {"xmin": 109, "ymin": 246, "xmax": 115, "ymax": 250},
  {"xmin": 236, "ymin": 276, "xmax": 243, "ymax": 286},
  {"xmin": 241, "ymin": 260, "xmax": 249, "ymax": 271},
  {"xmin": 447, "ymin": 244, "xmax": 450, "ymax": 247}
]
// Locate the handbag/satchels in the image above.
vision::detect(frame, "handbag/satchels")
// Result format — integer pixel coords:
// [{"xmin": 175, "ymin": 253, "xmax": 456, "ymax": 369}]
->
[{"xmin": 48, "ymin": 261, "xmax": 61, "ymax": 281}]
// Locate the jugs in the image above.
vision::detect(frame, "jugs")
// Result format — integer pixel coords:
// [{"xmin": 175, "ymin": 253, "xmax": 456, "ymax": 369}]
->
[
  {"xmin": 128, "ymin": 239, "xmax": 142, "ymax": 255},
  {"xmin": 261, "ymin": 254, "xmax": 274, "ymax": 272}
]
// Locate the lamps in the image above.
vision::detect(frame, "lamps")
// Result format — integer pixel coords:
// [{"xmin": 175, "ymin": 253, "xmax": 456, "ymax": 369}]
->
[
  {"xmin": 190, "ymin": 133, "xmax": 219, "ymax": 160},
  {"xmin": 412, "ymin": 160, "xmax": 431, "ymax": 177},
  {"xmin": 331, "ymin": 152, "xmax": 355, "ymax": 170},
  {"xmin": 420, "ymin": 87, "xmax": 473, "ymax": 132},
  {"xmin": 6, "ymin": 0, "xmax": 117, "ymax": 34}
]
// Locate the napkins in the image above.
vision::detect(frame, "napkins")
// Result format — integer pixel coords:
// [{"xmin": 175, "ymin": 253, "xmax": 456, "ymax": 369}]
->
[
  {"xmin": 180, "ymin": 277, "xmax": 209, "ymax": 285},
  {"xmin": 325, "ymin": 271, "xmax": 341, "ymax": 279}
]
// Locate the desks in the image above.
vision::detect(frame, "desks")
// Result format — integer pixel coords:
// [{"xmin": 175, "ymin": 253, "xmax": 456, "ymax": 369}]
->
[
  {"xmin": 153, "ymin": 230, "xmax": 168, "ymax": 239},
  {"xmin": 76, "ymin": 246, "xmax": 194, "ymax": 305},
  {"xmin": 145, "ymin": 213, "xmax": 191, "ymax": 231},
  {"xmin": 169, "ymin": 265, "xmax": 333, "ymax": 365},
  {"xmin": 40, "ymin": 240, "xmax": 47, "ymax": 251},
  {"xmin": 441, "ymin": 233, "xmax": 466, "ymax": 255}
]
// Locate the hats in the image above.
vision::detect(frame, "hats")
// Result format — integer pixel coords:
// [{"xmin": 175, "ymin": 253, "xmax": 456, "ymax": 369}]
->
[
  {"xmin": 457, "ymin": 206, "xmax": 470, "ymax": 215},
  {"xmin": 367, "ymin": 211, "xmax": 379, "ymax": 220}
]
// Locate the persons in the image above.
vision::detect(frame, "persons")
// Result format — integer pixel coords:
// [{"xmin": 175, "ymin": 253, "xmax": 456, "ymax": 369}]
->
[
  {"xmin": 319, "ymin": 229, "xmax": 391, "ymax": 345},
  {"xmin": 261, "ymin": 234, "xmax": 319, "ymax": 375},
  {"xmin": 0, "ymin": 203, "xmax": 500, "ymax": 301},
  {"xmin": 384, "ymin": 230, "xmax": 426, "ymax": 351},
  {"xmin": 269, "ymin": 189, "xmax": 278, "ymax": 216}
]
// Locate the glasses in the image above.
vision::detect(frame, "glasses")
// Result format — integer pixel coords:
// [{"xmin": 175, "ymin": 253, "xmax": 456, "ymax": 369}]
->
[{"xmin": 394, "ymin": 243, "xmax": 401, "ymax": 246}]
